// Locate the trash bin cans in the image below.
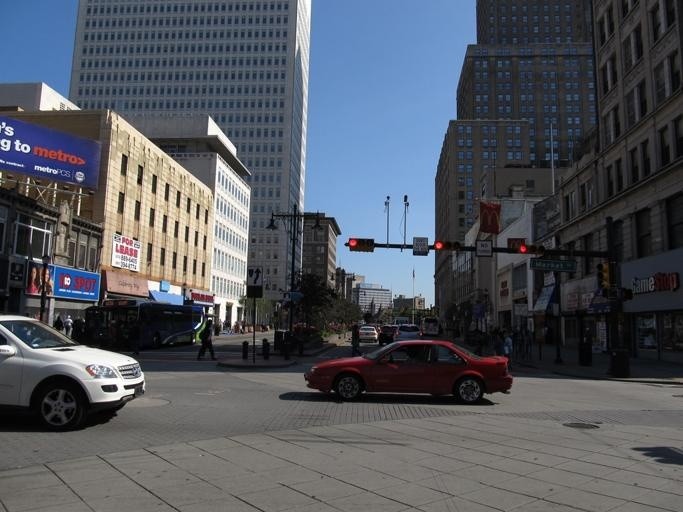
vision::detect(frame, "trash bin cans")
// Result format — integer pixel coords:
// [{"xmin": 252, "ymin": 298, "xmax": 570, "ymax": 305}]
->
[
  {"xmin": 615, "ymin": 350, "xmax": 630, "ymax": 378},
  {"xmin": 579, "ymin": 333, "xmax": 592, "ymax": 366}
]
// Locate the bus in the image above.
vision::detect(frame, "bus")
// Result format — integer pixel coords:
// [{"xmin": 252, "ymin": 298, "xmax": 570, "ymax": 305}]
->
[
  {"xmin": 100, "ymin": 299, "xmax": 208, "ymax": 349},
  {"xmin": 421, "ymin": 317, "xmax": 439, "ymax": 335}
]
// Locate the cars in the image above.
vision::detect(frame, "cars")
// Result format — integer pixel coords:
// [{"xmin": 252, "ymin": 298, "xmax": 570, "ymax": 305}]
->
[
  {"xmin": 304, "ymin": 343, "xmax": 513, "ymax": 404},
  {"xmin": 360, "ymin": 324, "xmax": 422, "ymax": 348},
  {"xmin": 1, "ymin": 314, "xmax": 145, "ymax": 430}
]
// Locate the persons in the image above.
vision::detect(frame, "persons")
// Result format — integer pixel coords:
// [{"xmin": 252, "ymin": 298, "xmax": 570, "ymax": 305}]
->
[
  {"xmin": 63, "ymin": 314, "xmax": 74, "ymax": 336},
  {"xmin": 213, "ymin": 318, "xmax": 246, "ymax": 336},
  {"xmin": 350, "ymin": 324, "xmax": 363, "ymax": 357},
  {"xmin": 38, "ymin": 267, "xmax": 52, "ymax": 295},
  {"xmin": 491, "ymin": 324, "xmax": 533, "ymax": 371},
  {"xmin": 25, "ymin": 266, "xmax": 42, "ymax": 293},
  {"xmin": 196, "ymin": 319, "xmax": 217, "ymax": 361},
  {"xmin": 55, "ymin": 316, "xmax": 64, "ymax": 331}
]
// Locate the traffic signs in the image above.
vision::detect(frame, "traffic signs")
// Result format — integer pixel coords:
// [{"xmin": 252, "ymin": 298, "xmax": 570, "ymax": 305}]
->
[{"xmin": 529, "ymin": 259, "xmax": 576, "ymax": 273}]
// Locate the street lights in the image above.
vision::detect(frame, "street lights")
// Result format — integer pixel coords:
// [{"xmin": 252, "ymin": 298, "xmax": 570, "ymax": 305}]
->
[
  {"xmin": 264, "ymin": 204, "xmax": 324, "ymax": 329},
  {"xmin": 330, "ymin": 270, "xmax": 365, "ymax": 304}
]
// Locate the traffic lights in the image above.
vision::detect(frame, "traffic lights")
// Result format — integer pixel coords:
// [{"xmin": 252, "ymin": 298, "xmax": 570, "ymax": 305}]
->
[
  {"xmin": 433, "ymin": 241, "xmax": 463, "ymax": 251},
  {"xmin": 348, "ymin": 238, "xmax": 375, "ymax": 253},
  {"xmin": 596, "ymin": 263, "xmax": 610, "ymax": 290},
  {"xmin": 520, "ymin": 243, "xmax": 546, "ymax": 255}
]
